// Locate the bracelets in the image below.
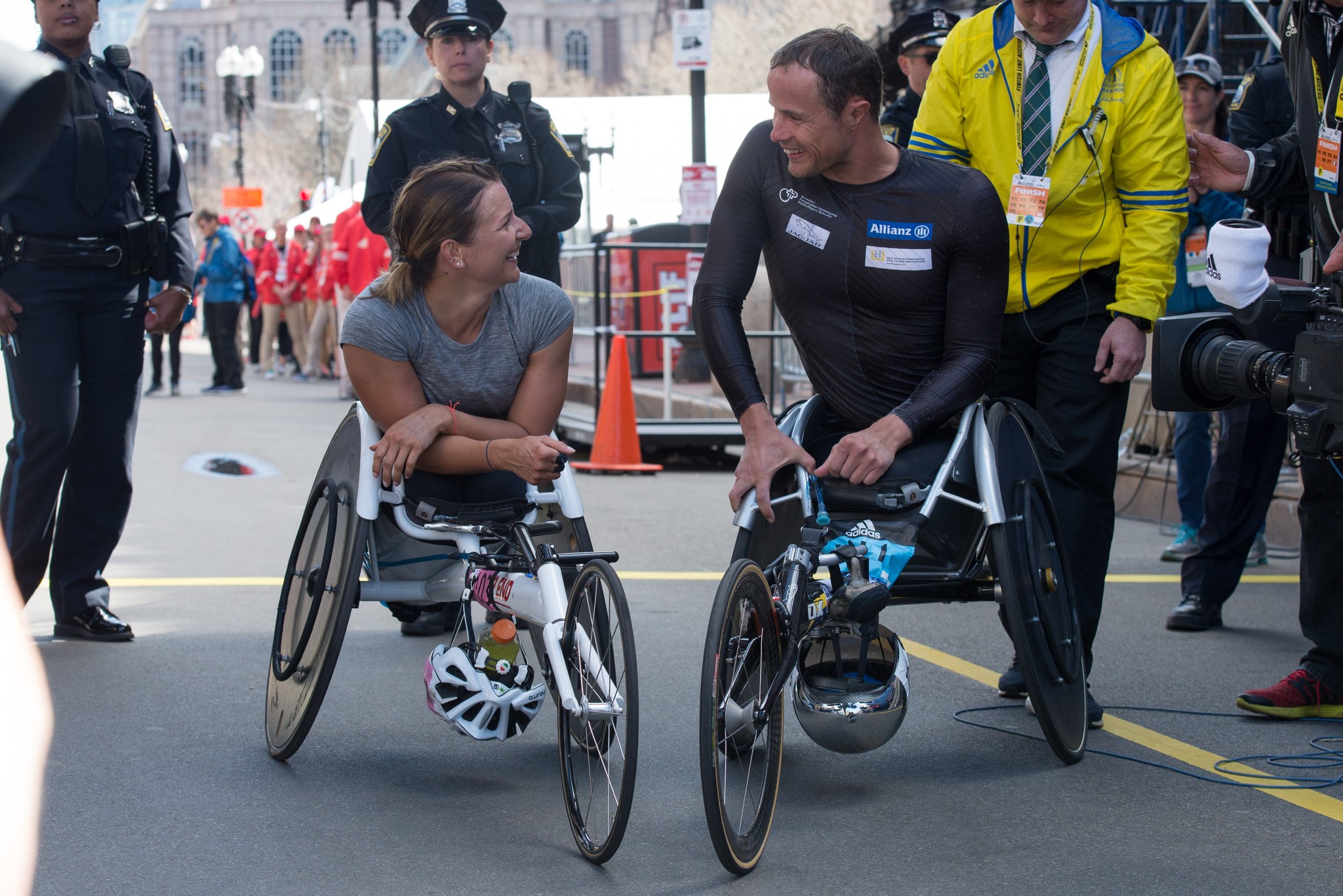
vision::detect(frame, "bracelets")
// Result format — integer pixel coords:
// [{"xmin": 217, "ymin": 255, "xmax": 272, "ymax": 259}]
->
[
  {"xmin": 445, "ymin": 400, "xmax": 459, "ymax": 435},
  {"xmin": 486, "ymin": 440, "xmax": 499, "ymax": 471}
]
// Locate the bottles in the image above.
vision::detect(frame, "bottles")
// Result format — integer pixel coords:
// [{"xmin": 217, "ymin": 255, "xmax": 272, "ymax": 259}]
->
[{"xmin": 477, "ymin": 619, "xmax": 520, "ymax": 671}]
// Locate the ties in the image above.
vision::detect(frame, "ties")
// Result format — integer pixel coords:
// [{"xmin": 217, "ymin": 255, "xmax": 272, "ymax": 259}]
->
[
  {"xmin": 460, "ymin": 106, "xmax": 492, "ymax": 164},
  {"xmin": 69, "ymin": 55, "xmax": 110, "ymax": 228},
  {"xmin": 1022, "ymin": 30, "xmax": 1073, "ymax": 178}
]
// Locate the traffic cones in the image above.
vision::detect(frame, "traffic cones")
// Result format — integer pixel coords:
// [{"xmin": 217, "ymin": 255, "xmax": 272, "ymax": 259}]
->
[{"xmin": 567, "ymin": 333, "xmax": 666, "ymax": 476}]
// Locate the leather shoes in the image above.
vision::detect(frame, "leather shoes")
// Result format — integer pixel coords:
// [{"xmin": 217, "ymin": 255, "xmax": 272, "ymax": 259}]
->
[
  {"xmin": 1166, "ymin": 593, "xmax": 1224, "ymax": 631},
  {"xmin": 54, "ymin": 604, "xmax": 136, "ymax": 641}
]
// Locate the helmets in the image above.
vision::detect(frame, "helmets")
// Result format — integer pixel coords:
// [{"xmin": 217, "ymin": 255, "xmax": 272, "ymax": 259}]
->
[
  {"xmin": 424, "ymin": 643, "xmax": 547, "ymax": 743},
  {"xmin": 789, "ymin": 621, "xmax": 910, "ymax": 754}
]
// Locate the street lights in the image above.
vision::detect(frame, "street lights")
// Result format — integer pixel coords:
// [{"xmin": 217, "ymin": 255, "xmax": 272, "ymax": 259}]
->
[{"xmin": 213, "ymin": 44, "xmax": 265, "ymax": 247}]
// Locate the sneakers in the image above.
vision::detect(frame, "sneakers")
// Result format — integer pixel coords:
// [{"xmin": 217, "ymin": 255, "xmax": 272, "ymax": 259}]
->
[
  {"xmin": 1160, "ymin": 522, "xmax": 1201, "ymax": 562},
  {"xmin": 1244, "ymin": 532, "xmax": 1268, "ymax": 567},
  {"xmin": 1236, "ymin": 668, "xmax": 1343, "ymax": 720}
]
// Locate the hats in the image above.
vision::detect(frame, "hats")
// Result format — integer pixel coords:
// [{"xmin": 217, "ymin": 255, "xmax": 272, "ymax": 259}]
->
[
  {"xmin": 409, "ymin": 1, "xmax": 507, "ymax": 37},
  {"xmin": 218, "ymin": 215, "xmax": 230, "ymax": 225},
  {"xmin": 255, "ymin": 228, "xmax": 266, "ymax": 238},
  {"xmin": 1173, "ymin": 52, "xmax": 1225, "ymax": 88},
  {"xmin": 887, "ymin": 5, "xmax": 961, "ymax": 55},
  {"xmin": 294, "ymin": 224, "xmax": 305, "ymax": 233}
]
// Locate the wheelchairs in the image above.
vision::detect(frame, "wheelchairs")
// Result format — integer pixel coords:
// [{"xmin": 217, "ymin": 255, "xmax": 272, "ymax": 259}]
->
[
  {"xmin": 262, "ymin": 393, "xmax": 640, "ymax": 864},
  {"xmin": 697, "ymin": 387, "xmax": 1090, "ymax": 878}
]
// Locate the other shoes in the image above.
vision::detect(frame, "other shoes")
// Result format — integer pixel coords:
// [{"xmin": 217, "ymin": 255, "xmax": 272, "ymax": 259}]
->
[
  {"xmin": 202, "ymin": 381, "xmax": 245, "ymax": 393},
  {"xmin": 250, "ymin": 352, "xmax": 342, "ymax": 383},
  {"xmin": 144, "ymin": 382, "xmax": 162, "ymax": 395},
  {"xmin": 998, "ymin": 650, "xmax": 1030, "ymax": 697},
  {"xmin": 1024, "ymin": 686, "xmax": 1104, "ymax": 731},
  {"xmin": 171, "ymin": 383, "xmax": 179, "ymax": 395},
  {"xmin": 401, "ymin": 608, "xmax": 468, "ymax": 634}
]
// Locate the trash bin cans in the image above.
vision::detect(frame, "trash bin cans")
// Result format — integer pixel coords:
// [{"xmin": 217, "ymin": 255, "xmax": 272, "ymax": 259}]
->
[{"xmin": 604, "ymin": 222, "xmax": 687, "ymax": 378}]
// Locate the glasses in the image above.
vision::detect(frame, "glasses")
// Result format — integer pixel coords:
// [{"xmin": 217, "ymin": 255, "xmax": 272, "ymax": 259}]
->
[
  {"xmin": 907, "ymin": 50, "xmax": 938, "ymax": 66},
  {"xmin": 1174, "ymin": 58, "xmax": 1211, "ymax": 72}
]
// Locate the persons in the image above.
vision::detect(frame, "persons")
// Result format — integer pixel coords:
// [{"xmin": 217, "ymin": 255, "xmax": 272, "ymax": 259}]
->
[
  {"xmin": 342, "ymin": 159, "xmax": 574, "ymax": 499},
  {"xmin": 877, "ymin": 0, "xmax": 1343, "ymax": 720},
  {"xmin": 690, "ymin": 26, "xmax": 1011, "ymax": 568},
  {"xmin": 359, "ymin": 0, "xmax": 583, "ymax": 634},
  {"xmin": 145, "ymin": 199, "xmax": 391, "ymax": 401},
  {"xmin": 0, "ymin": 0, "xmax": 193, "ymax": 642}
]
